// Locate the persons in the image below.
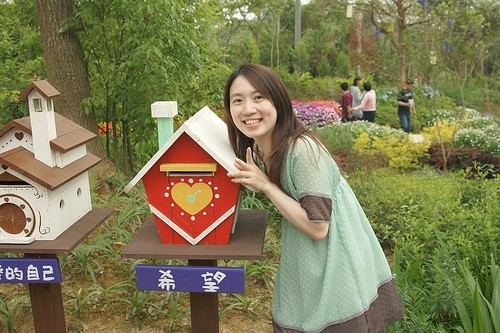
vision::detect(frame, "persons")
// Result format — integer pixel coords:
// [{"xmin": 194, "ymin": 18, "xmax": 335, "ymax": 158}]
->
[
  {"xmin": 223, "ymin": 63, "xmax": 404, "ymax": 333},
  {"xmin": 340, "ymin": 77, "xmax": 416, "ymax": 133}
]
